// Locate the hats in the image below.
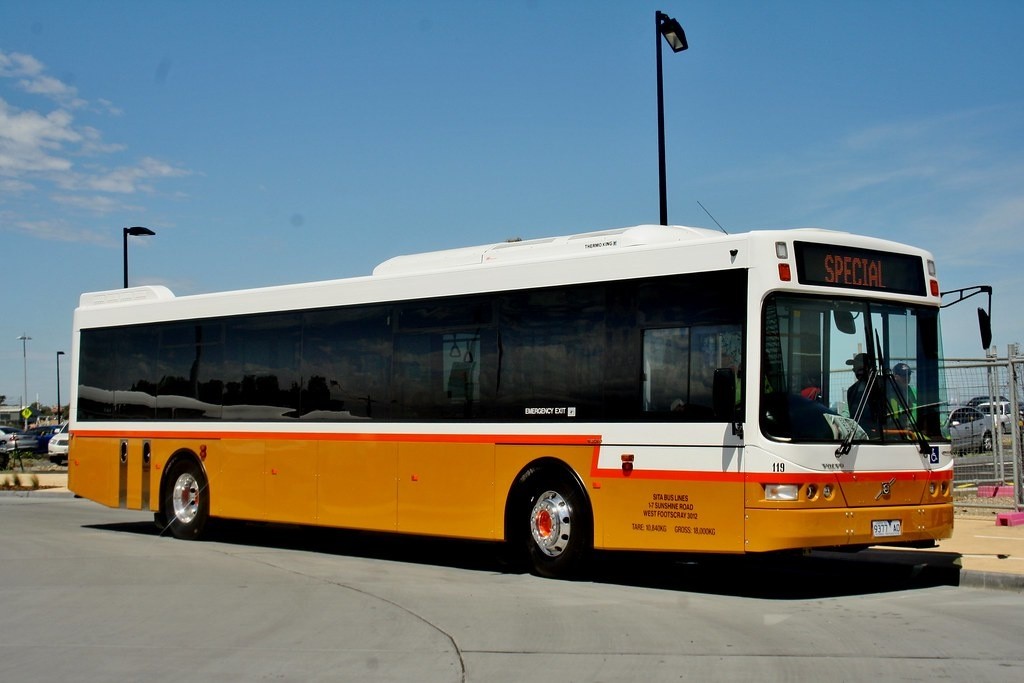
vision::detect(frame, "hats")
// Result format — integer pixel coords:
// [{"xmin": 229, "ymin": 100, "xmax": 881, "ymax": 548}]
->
[
  {"xmin": 893, "ymin": 363, "xmax": 913, "ymax": 375},
  {"xmin": 846, "ymin": 353, "xmax": 869, "ymax": 365}
]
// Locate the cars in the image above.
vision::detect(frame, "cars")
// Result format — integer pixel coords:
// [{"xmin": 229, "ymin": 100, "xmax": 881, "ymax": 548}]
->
[
  {"xmin": 0, "ymin": 422, "xmax": 70, "ymax": 468},
  {"xmin": 940, "ymin": 405, "xmax": 992, "ymax": 454},
  {"xmin": 966, "ymin": 395, "xmax": 1024, "ymax": 434}
]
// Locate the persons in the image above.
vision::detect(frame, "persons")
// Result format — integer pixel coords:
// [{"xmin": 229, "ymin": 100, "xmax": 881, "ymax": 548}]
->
[{"xmin": 845, "ymin": 353, "xmax": 916, "ymax": 441}]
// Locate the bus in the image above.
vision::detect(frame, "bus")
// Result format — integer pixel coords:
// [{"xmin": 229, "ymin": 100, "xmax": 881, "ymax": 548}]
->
[{"xmin": 69, "ymin": 224, "xmax": 993, "ymax": 578}]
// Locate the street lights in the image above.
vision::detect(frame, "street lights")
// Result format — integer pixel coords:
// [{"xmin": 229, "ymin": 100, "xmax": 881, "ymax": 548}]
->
[
  {"xmin": 654, "ymin": 12, "xmax": 689, "ymax": 224},
  {"xmin": 57, "ymin": 351, "xmax": 65, "ymax": 425},
  {"xmin": 16, "ymin": 332, "xmax": 32, "ymax": 429},
  {"xmin": 123, "ymin": 227, "xmax": 156, "ymax": 288}
]
